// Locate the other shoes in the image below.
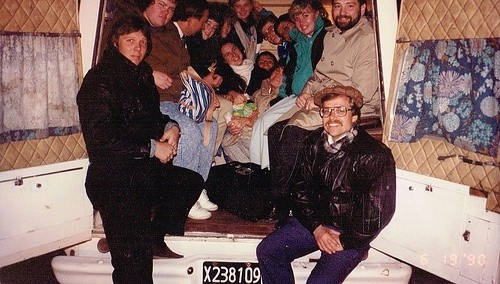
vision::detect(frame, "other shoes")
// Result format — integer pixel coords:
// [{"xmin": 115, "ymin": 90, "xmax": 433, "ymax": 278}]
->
[
  {"xmin": 197, "ymin": 189, "xmax": 218, "ymax": 211},
  {"xmin": 152, "ymin": 242, "xmax": 183, "ymax": 259},
  {"xmin": 188, "ymin": 202, "xmax": 211, "ymax": 220}
]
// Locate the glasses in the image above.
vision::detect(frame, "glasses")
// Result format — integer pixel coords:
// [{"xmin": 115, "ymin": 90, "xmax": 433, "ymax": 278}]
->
[
  {"xmin": 319, "ymin": 106, "xmax": 353, "ymax": 118},
  {"xmin": 263, "ymin": 27, "xmax": 274, "ymax": 41}
]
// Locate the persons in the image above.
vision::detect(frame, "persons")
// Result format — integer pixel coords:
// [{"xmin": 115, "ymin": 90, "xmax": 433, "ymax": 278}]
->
[
  {"xmin": 239, "ymin": 0, "xmax": 333, "ymax": 170},
  {"xmin": 257, "ymin": 85, "xmax": 396, "ymax": 284},
  {"xmin": 141, "ymin": 0, "xmax": 217, "ymax": 220},
  {"xmin": 264, "ymin": 0, "xmax": 379, "ymax": 231},
  {"xmin": 76, "ymin": 14, "xmax": 204, "ymax": 284},
  {"xmin": 172, "ymin": 0, "xmax": 297, "ymax": 164}
]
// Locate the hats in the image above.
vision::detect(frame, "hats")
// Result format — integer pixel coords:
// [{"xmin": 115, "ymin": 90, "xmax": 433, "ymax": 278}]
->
[{"xmin": 314, "ymin": 86, "xmax": 363, "ymax": 108}]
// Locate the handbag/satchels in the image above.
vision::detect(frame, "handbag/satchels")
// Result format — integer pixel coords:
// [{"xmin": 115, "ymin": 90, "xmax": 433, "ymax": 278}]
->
[{"xmin": 178, "ymin": 69, "xmax": 217, "ymax": 124}]
